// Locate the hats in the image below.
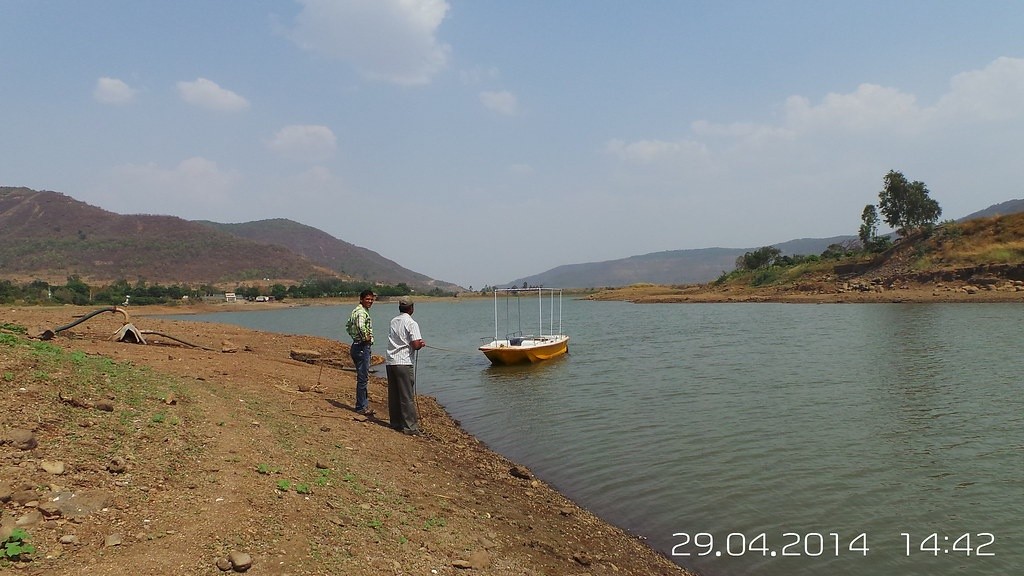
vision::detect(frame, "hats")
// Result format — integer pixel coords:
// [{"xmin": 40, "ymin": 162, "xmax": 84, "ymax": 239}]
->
[{"xmin": 398, "ymin": 296, "xmax": 414, "ymax": 307}]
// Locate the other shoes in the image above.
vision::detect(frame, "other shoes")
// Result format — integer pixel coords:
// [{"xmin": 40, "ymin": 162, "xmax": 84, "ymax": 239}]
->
[{"xmin": 362, "ymin": 408, "xmax": 377, "ymax": 414}]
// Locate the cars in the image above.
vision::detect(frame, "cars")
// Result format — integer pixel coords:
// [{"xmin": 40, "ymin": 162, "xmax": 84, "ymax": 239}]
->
[{"xmin": 256, "ymin": 296, "xmax": 269, "ymax": 302}]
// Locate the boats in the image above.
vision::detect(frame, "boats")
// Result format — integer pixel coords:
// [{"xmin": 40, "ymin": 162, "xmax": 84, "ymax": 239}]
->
[{"xmin": 478, "ymin": 288, "xmax": 570, "ymax": 364}]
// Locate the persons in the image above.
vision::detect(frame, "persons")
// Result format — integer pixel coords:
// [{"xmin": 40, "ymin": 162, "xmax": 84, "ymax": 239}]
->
[
  {"xmin": 386, "ymin": 295, "xmax": 425, "ymax": 436},
  {"xmin": 345, "ymin": 290, "xmax": 378, "ymax": 415}
]
